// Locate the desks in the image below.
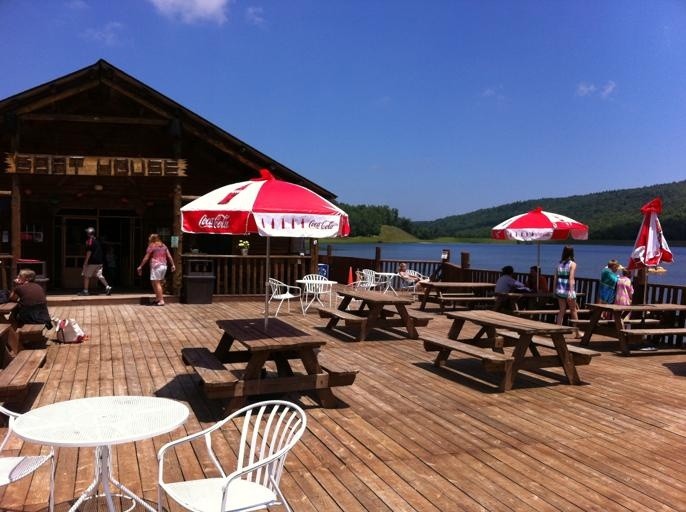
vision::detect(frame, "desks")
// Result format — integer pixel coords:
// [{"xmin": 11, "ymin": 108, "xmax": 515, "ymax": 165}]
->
[
  {"xmin": 337, "ymin": 291, "xmax": 419, "ymax": 341},
  {"xmin": 8, "ymin": 395, "xmax": 190, "ymax": 512},
  {"xmin": 216, "ymin": 319, "xmax": 338, "ymax": 418}
]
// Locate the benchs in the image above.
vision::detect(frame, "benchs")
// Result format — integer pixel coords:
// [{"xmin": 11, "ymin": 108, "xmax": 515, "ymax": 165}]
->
[
  {"xmin": 316, "ymin": 306, "xmax": 367, "ymax": 340},
  {"xmin": 181, "ymin": 347, "xmax": 245, "ymax": 417},
  {"xmin": 381, "ymin": 305, "xmax": 433, "ymax": 338},
  {"xmin": 315, "ymin": 348, "xmax": 359, "ymax": 408},
  {"xmin": 0, "ymin": 301, "xmax": 52, "ymax": 403},
  {"xmin": 417, "ymin": 280, "xmax": 686, "ymax": 392}
]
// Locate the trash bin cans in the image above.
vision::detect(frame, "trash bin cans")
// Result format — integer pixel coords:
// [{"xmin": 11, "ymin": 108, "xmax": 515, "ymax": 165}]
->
[
  {"xmin": 182, "ymin": 259, "xmax": 216, "ymax": 304},
  {"xmin": 15, "ymin": 261, "xmax": 49, "ymax": 297}
]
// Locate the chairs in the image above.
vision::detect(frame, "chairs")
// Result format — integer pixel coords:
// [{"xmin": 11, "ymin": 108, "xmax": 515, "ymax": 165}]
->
[
  {"xmin": 0, "ymin": 404, "xmax": 58, "ymax": 510},
  {"xmin": 155, "ymin": 400, "xmax": 307, "ymax": 512},
  {"xmin": 342, "ymin": 268, "xmax": 430, "ymax": 296},
  {"xmin": 261, "ymin": 274, "xmax": 337, "ymax": 318}
]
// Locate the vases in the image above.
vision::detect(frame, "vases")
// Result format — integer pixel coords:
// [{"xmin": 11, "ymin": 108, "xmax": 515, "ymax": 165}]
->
[{"xmin": 241, "ymin": 249, "xmax": 248, "ymax": 256}]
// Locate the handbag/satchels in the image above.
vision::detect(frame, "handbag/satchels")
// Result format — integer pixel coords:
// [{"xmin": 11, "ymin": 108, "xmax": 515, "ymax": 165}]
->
[{"xmin": 54, "ymin": 318, "xmax": 85, "ymax": 343}]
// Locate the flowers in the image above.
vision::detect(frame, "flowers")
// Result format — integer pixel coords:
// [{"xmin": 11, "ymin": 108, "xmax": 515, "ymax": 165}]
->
[{"xmin": 238, "ymin": 239, "xmax": 251, "ymax": 249}]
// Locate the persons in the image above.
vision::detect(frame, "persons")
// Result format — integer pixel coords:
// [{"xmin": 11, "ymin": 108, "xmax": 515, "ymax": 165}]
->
[
  {"xmin": 552, "ymin": 244, "xmax": 584, "ymax": 339},
  {"xmin": 397, "ymin": 261, "xmax": 428, "ymax": 293},
  {"xmin": 496, "ymin": 266, "xmax": 531, "ymax": 295},
  {"xmin": 598, "ymin": 259, "xmax": 621, "ymax": 320},
  {"xmin": 135, "ymin": 233, "xmax": 178, "ymax": 305},
  {"xmin": 617, "ymin": 266, "xmax": 634, "ymax": 322},
  {"xmin": 526, "ymin": 265, "xmax": 547, "ymax": 294},
  {"xmin": 4, "ymin": 269, "xmax": 52, "ymax": 357},
  {"xmin": 77, "ymin": 226, "xmax": 113, "ymax": 296}
]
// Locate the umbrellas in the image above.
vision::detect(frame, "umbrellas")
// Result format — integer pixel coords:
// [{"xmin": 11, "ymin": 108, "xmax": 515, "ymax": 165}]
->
[
  {"xmin": 491, "ymin": 206, "xmax": 590, "ymax": 302},
  {"xmin": 178, "ymin": 169, "xmax": 352, "ymax": 331},
  {"xmin": 628, "ymin": 194, "xmax": 675, "ymax": 321}
]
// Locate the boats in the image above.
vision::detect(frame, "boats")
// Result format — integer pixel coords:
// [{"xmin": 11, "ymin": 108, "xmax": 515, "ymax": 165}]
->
[{"xmin": 648, "ymin": 266, "xmax": 668, "ymax": 274}]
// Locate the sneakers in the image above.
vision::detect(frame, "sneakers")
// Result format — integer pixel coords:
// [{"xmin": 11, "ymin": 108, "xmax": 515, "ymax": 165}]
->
[
  {"xmin": 105, "ymin": 287, "xmax": 112, "ymax": 294},
  {"xmin": 78, "ymin": 291, "xmax": 89, "ymax": 295}
]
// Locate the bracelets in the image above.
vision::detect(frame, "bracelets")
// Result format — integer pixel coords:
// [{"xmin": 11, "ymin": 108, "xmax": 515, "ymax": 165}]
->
[{"xmin": 82, "ymin": 263, "xmax": 88, "ymax": 267}]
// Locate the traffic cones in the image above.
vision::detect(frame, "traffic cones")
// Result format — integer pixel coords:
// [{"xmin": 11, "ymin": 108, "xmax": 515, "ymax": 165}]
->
[{"xmin": 347, "ymin": 266, "xmax": 355, "ymax": 289}]
